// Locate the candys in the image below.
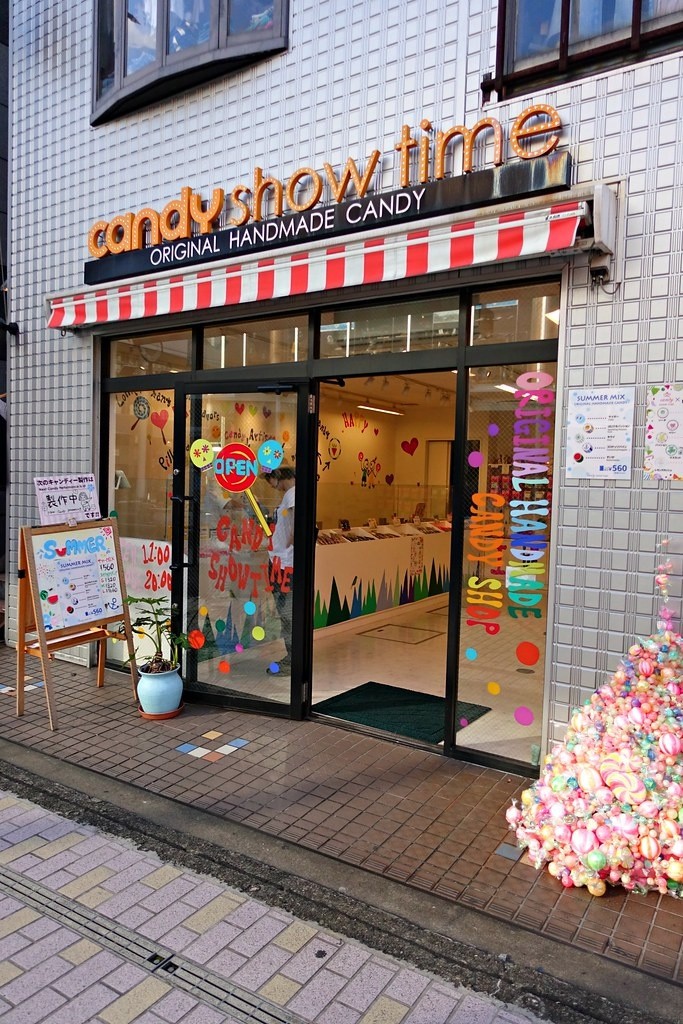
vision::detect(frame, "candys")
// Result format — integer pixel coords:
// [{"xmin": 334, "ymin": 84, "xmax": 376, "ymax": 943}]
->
[{"xmin": 506, "ymin": 539, "xmax": 683, "ymax": 899}]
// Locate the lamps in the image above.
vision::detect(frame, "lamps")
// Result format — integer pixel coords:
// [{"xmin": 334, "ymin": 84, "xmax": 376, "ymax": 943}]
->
[
  {"xmin": 478, "ymin": 367, "xmax": 490, "ymax": 378},
  {"xmin": 425, "ymin": 387, "xmax": 432, "ymax": 401},
  {"xmin": 401, "ymin": 380, "xmax": 410, "ymax": 396},
  {"xmin": 356, "ymin": 397, "xmax": 405, "ymax": 416},
  {"xmin": 382, "ymin": 376, "xmax": 389, "ymax": 392},
  {"xmin": 439, "ymin": 391, "xmax": 451, "ymax": 407},
  {"xmin": 365, "ymin": 377, "xmax": 376, "ymax": 387}
]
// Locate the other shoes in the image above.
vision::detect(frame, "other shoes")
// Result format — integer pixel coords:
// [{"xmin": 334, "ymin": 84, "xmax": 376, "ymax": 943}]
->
[{"xmin": 266, "ymin": 655, "xmax": 291, "ymax": 676}]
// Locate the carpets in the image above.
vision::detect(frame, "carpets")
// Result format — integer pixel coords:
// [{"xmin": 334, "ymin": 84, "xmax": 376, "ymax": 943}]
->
[{"xmin": 311, "ymin": 681, "xmax": 492, "ymax": 745}]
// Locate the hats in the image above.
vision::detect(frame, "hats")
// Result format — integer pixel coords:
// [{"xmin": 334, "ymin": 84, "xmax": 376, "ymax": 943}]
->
[{"xmin": 259, "ymin": 457, "xmax": 290, "ymax": 479}]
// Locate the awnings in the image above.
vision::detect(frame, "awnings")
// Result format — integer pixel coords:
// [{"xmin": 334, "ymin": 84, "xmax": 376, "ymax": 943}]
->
[{"xmin": 42, "ymin": 201, "xmax": 593, "ymax": 327}]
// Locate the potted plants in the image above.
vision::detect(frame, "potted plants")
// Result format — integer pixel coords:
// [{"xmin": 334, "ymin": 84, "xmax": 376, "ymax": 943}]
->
[{"xmin": 112, "ymin": 596, "xmax": 190, "ymax": 719}]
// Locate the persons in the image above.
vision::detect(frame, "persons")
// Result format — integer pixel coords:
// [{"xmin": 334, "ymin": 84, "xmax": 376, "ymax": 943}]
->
[{"xmin": 257, "ymin": 457, "xmax": 296, "ymax": 678}]
